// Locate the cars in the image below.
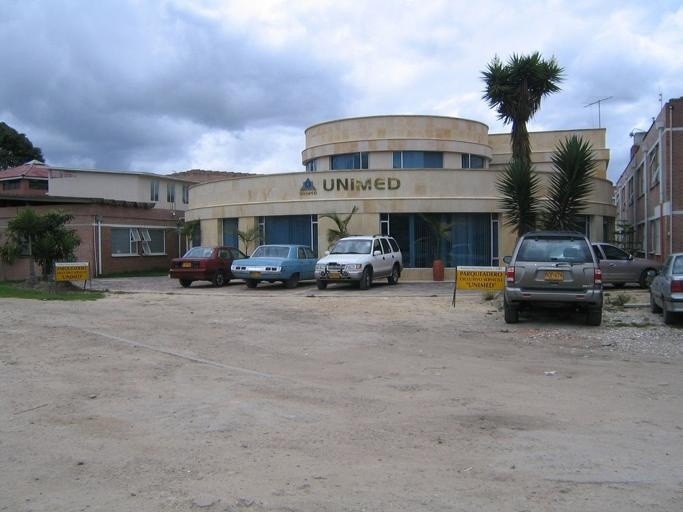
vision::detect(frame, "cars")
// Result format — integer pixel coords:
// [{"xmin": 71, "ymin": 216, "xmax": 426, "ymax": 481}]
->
[
  {"xmin": 169, "ymin": 246, "xmax": 249, "ymax": 287},
  {"xmin": 230, "ymin": 243, "xmax": 318, "ymax": 289},
  {"xmin": 502, "ymin": 230, "xmax": 603, "ymax": 326},
  {"xmin": 649, "ymin": 253, "xmax": 682, "ymax": 325},
  {"xmin": 590, "ymin": 242, "xmax": 660, "ymax": 289}
]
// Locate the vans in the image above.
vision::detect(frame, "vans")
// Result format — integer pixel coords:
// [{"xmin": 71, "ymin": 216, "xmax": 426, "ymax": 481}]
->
[{"xmin": 313, "ymin": 234, "xmax": 403, "ymax": 289}]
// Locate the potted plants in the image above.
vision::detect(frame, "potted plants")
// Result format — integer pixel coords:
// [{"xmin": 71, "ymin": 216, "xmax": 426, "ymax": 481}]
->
[{"xmin": 417, "ymin": 210, "xmax": 465, "ymax": 281}]
